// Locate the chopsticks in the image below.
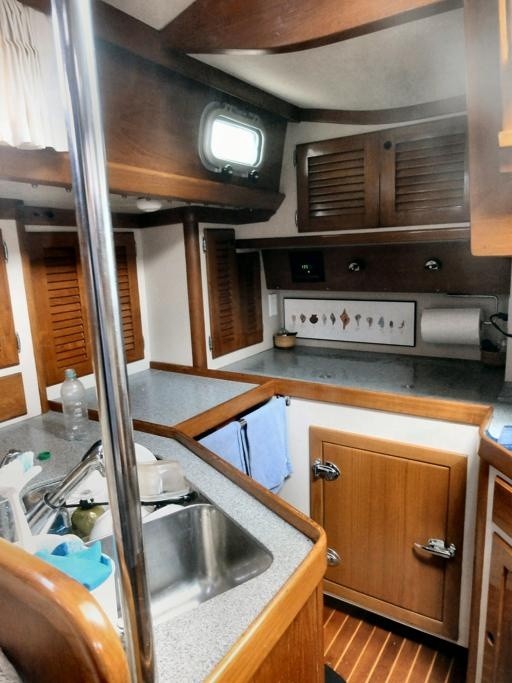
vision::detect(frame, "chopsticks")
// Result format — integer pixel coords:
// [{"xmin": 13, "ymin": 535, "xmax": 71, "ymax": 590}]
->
[{"xmin": 95, "ymin": 441, "xmax": 162, "ymax": 509}]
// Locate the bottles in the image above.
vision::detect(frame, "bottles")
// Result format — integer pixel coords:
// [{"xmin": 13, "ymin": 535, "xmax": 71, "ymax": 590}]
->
[{"xmin": 61, "ymin": 367, "xmax": 90, "ymax": 442}]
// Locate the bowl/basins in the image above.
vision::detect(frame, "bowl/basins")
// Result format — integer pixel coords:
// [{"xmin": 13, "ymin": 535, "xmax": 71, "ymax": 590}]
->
[
  {"xmin": 88, "ymin": 505, "xmax": 153, "ymax": 540},
  {"xmin": 274, "ymin": 333, "xmax": 296, "ymax": 349}
]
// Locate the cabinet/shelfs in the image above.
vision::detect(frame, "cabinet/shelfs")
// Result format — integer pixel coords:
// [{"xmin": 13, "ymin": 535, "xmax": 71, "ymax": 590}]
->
[
  {"xmin": 481, "ymin": 475, "xmax": 512, "ymax": 682},
  {"xmin": 308, "ymin": 429, "xmax": 471, "ymax": 644},
  {"xmin": 289, "ymin": 109, "xmax": 472, "ymax": 232},
  {"xmin": 24, "ymin": 230, "xmax": 143, "ymax": 386},
  {"xmin": 202, "ymin": 225, "xmax": 269, "ymax": 358}
]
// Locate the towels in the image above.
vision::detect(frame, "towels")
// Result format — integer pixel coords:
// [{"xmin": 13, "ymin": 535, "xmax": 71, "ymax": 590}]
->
[
  {"xmin": 240, "ymin": 394, "xmax": 292, "ymax": 495},
  {"xmin": 197, "ymin": 418, "xmax": 248, "ymax": 475}
]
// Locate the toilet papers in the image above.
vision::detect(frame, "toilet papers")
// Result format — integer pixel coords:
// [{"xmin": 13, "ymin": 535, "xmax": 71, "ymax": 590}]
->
[{"xmin": 420, "ymin": 307, "xmax": 483, "ymax": 347}]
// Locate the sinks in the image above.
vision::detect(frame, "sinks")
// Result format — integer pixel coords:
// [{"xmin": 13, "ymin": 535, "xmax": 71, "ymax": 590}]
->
[
  {"xmin": 19, "ymin": 451, "xmax": 197, "ymax": 544},
  {"xmin": 83, "ymin": 504, "xmax": 274, "ymax": 635}
]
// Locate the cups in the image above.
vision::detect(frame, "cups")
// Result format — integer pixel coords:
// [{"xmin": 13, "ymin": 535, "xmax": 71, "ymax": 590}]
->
[{"xmin": 136, "ymin": 459, "xmax": 184, "ymax": 496}]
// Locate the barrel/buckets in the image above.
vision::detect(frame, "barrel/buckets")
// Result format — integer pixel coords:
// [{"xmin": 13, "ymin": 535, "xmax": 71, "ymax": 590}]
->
[{"xmin": 14, "ymin": 533, "xmax": 117, "ymax": 633}]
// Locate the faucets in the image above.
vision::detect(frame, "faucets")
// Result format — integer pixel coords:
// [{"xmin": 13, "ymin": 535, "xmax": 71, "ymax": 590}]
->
[{"xmin": 9, "ymin": 438, "xmax": 108, "ymax": 543}]
